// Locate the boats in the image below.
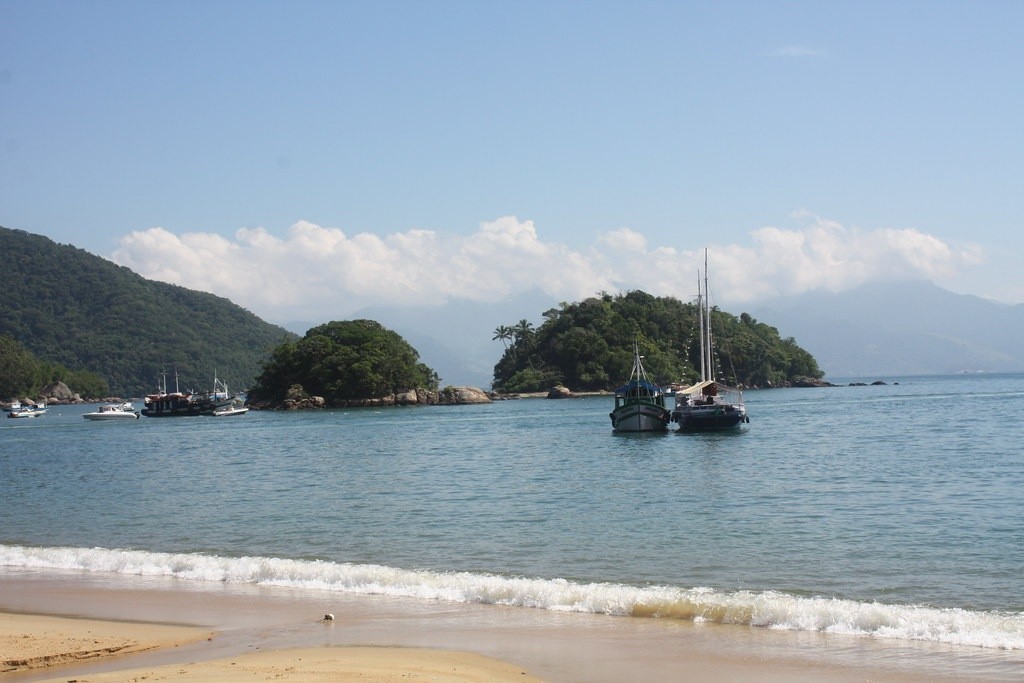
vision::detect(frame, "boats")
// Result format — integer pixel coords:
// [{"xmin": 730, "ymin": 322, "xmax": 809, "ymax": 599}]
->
[
  {"xmin": 212, "ymin": 405, "xmax": 251, "ymax": 417},
  {"xmin": 610, "ymin": 339, "xmax": 672, "ymax": 433},
  {"xmin": 140, "ymin": 364, "xmax": 243, "ymax": 417},
  {"xmin": 98, "ymin": 400, "xmax": 135, "ymax": 411},
  {"xmin": 82, "ymin": 405, "xmax": 140, "ymax": 420},
  {"xmin": 6, "ymin": 402, "xmax": 49, "ymax": 418}
]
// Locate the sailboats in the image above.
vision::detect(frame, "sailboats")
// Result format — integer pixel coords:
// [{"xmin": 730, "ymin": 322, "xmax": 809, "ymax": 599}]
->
[{"xmin": 669, "ymin": 246, "xmax": 751, "ymax": 428}]
